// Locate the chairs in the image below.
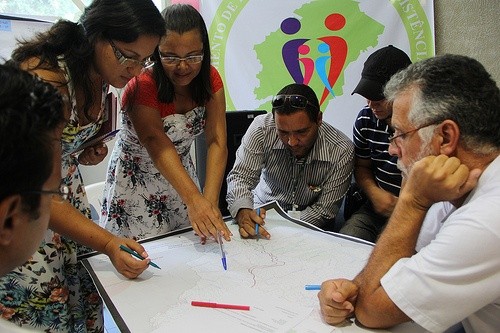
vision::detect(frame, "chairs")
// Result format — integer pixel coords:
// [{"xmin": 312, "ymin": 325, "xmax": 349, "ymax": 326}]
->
[{"xmin": 84, "ymin": 181, "xmax": 106, "ymax": 228}]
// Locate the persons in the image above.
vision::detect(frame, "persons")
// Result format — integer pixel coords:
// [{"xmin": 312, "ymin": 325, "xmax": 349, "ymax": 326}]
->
[
  {"xmin": 339, "ymin": 44, "xmax": 413, "ymax": 242},
  {"xmin": 0, "ymin": 0, "xmax": 167, "ymax": 333},
  {"xmin": 317, "ymin": 53, "xmax": 500, "ymax": 333},
  {"xmin": 99, "ymin": 4, "xmax": 233, "ymax": 244},
  {"xmin": 227, "ymin": 83, "xmax": 356, "ymax": 239},
  {"xmin": 0, "ymin": 65, "xmax": 66, "ymax": 333}
]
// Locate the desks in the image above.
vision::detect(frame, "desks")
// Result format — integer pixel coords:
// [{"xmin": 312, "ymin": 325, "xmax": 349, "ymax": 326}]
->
[{"xmin": 76, "ymin": 201, "xmax": 464, "ymax": 333}]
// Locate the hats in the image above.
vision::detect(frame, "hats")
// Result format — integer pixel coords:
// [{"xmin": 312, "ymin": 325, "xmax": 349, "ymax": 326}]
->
[{"xmin": 351, "ymin": 44, "xmax": 412, "ymax": 101}]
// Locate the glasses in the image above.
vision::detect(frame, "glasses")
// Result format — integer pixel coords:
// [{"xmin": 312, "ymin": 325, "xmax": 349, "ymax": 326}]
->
[
  {"xmin": 108, "ymin": 38, "xmax": 156, "ymax": 69},
  {"xmin": 272, "ymin": 95, "xmax": 315, "ymax": 111},
  {"xmin": 15, "ymin": 184, "xmax": 69, "ymax": 202},
  {"xmin": 388, "ymin": 119, "xmax": 448, "ymax": 149},
  {"xmin": 158, "ymin": 49, "xmax": 204, "ymax": 64}
]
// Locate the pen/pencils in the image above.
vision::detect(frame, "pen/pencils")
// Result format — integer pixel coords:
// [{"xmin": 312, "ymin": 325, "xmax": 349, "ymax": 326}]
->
[
  {"xmin": 191, "ymin": 301, "xmax": 250, "ymax": 311},
  {"xmin": 216, "ymin": 231, "xmax": 227, "ymax": 270},
  {"xmin": 254, "ymin": 207, "xmax": 260, "ymax": 238},
  {"xmin": 119, "ymin": 244, "xmax": 162, "ymax": 270},
  {"xmin": 305, "ymin": 285, "xmax": 321, "ymax": 290}
]
